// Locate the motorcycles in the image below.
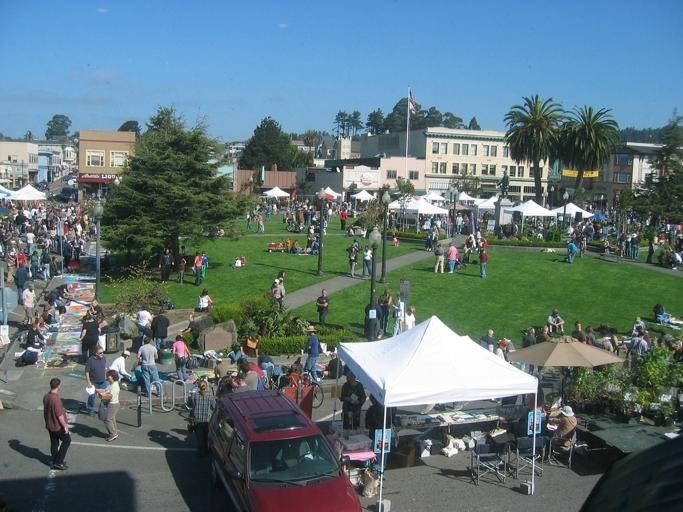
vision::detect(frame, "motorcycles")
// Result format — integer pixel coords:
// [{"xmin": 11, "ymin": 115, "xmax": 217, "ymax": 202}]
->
[{"xmin": 285, "ymin": 216, "xmax": 304, "ymax": 232}]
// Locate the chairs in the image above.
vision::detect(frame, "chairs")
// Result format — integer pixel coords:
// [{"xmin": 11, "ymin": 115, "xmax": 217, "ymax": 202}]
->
[
  {"xmin": 469, "ymin": 443, "xmax": 506, "ymax": 485},
  {"xmin": 280, "ymin": 433, "xmax": 312, "ymax": 468},
  {"xmin": 508, "ymin": 436, "xmax": 544, "ymax": 479},
  {"xmin": 548, "ymin": 429, "xmax": 576, "ymax": 468}
]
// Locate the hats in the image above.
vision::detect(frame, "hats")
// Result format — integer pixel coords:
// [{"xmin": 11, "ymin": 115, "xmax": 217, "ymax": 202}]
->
[
  {"xmin": 305, "ymin": 325, "xmax": 318, "ymax": 332},
  {"xmin": 85, "ymin": 385, "xmax": 95, "ymax": 395},
  {"xmin": 123, "ymin": 351, "xmax": 130, "ymax": 356},
  {"xmin": 559, "ymin": 406, "xmax": 574, "ymax": 417}
]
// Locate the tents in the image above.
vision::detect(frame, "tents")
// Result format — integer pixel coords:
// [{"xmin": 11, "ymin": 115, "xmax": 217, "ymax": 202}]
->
[{"xmin": 263, "ymin": 186, "xmax": 592, "ymax": 234}]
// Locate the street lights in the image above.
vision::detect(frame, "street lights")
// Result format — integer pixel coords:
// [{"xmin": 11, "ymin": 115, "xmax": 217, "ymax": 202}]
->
[
  {"xmin": 366, "ymin": 227, "xmax": 381, "ymax": 343},
  {"xmin": 549, "ymin": 186, "xmax": 555, "ymax": 210},
  {"xmin": 379, "ymin": 190, "xmax": 390, "ymax": 282},
  {"xmin": 561, "ymin": 191, "xmax": 569, "ymax": 232},
  {"xmin": 600, "ymin": 194, "xmax": 604, "ymax": 210},
  {"xmin": 449, "ymin": 186, "xmax": 458, "ymax": 249},
  {"xmin": 92, "ymin": 202, "xmax": 103, "ymax": 305},
  {"xmin": 638, "ymin": 186, "xmax": 664, "ymax": 216},
  {"xmin": 314, "ymin": 187, "xmax": 326, "ymax": 276}
]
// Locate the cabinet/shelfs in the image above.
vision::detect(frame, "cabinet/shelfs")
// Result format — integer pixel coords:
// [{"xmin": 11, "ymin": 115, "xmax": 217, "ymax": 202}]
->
[{"xmin": 328, "ymin": 403, "xmax": 527, "ymax": 479}]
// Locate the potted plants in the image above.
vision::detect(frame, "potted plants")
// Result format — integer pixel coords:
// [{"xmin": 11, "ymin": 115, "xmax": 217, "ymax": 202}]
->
[{"xmin": 564, "ymin": 337, "xmax": 683, "ymax": 425}]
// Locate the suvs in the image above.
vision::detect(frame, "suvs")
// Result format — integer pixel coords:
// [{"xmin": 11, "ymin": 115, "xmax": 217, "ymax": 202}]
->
[
  {"xmin": 205, "ymin": 390, "xmax": 362, "ymax": 511},
  {"xmin": 59, "ymin": 187, "xmax": 77, "ymax": 199}
]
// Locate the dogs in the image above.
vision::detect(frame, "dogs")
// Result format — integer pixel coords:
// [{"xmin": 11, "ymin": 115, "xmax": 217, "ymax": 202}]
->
[{"xmin": 540, "ymin": 248, "xmax": 555, "ymax": 253}]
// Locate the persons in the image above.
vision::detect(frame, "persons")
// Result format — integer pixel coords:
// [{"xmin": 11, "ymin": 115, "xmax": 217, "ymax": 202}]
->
[
  {"xmin": 366, "ymin": 391, "xmax": 389, "ymax": 474},
  {"xmin": 0, "ymin": 182, "xmax": 101, "ymax": 366},
  {"xmin": 79, "ymin": 289, "xmax": 421, "ymax": 443},
  {"xmin": 469, "ymin": 302, "xmax": 682, "ymax": 453},
  {"xmin": 161, "ymin": 198, "xmax": 681, "ymax": 284},
  {"xmin": 42, "ymin": 377, "xmax": 71, "ymax": 471}
]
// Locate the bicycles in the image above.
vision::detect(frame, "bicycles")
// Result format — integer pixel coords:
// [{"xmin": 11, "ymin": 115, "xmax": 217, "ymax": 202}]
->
[{"xmin": 277, "ymin": 369, "xmax": 323, "ymax": 408}]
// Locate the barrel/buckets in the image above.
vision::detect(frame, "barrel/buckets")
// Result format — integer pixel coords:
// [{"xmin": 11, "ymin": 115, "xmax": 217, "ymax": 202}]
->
[{"xmin": 159, "ymin": 340, "xmax": 175, "ymax": 365}]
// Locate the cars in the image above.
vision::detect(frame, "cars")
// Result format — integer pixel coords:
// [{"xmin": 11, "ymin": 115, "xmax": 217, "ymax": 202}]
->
[
  {"xmin": 11, "ymin": 187, "xmax": 20, "ymax": 191},
  {"xmin": 33, "ymin": 183, "xmax": 41, "ymax": 191},
  {"xmin": 71, "ymin": 176, "xmax": 77, "ymax": 183},
  {"xmin": 74, "ymin": 169, "xmax": 78, "ymax": 173},
  {"xmin": 68, "ymin": 171, "xmax": 73, "ymax": 175},
  {"xmin": 63, "ymin": 175, "xmax": 69, "ymax": 181},
  {"xmin": 39, "ymin": 181, "xmax": 48, "ymax": 190}
]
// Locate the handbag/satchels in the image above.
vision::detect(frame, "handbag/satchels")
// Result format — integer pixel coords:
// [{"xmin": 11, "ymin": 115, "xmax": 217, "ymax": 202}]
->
[
  {"xmin": 175, "ymin": 354, "xmax": 186, "ymax": 368},
  {"xmin": 98, "ymin": 399, "xmax": 108, "ymax": 421}
]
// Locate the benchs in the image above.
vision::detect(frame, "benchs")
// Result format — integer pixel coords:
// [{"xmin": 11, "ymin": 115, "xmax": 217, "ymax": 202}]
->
[{"xmin": 599, "ymin": 252, "xmax": 623, "ymax": 264}]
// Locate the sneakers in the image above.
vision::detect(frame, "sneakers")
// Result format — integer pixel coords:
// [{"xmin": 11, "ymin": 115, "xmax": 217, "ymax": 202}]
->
[
  {"xmin": 108, "ymin": 434, "xmax": 118, "ymax": 442},
  {"xmin": 53, "ymin": 462, "xmax": 67, "ymax": 470}
]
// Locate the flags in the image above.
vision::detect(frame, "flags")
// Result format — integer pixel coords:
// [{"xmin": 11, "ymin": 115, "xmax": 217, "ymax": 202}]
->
[{"xmin": 408, "ymin": 88, "xmax": 415, "ymax": 117}]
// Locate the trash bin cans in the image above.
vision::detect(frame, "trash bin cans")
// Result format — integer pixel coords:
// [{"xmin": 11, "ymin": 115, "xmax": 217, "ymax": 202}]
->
[{"xmin": 101, "ymin": 325, "xmax": 120, "ymax": 354}]
